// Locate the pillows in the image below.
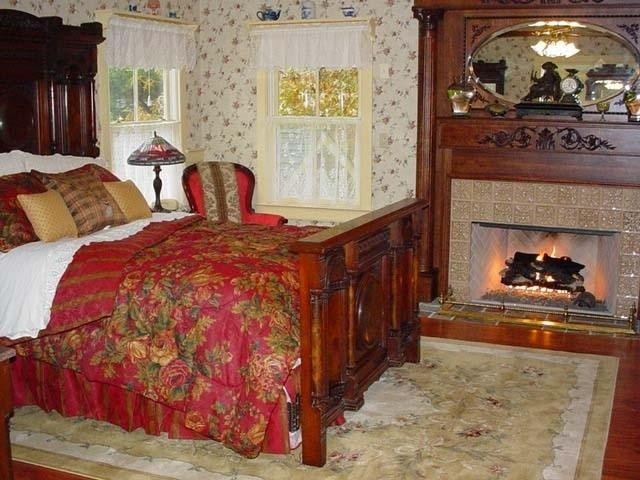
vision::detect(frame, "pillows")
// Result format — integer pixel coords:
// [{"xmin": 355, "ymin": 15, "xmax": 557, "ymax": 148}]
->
[{"xmin": 0, "ymin": 148, "xmax": 153, "ymax": 253}]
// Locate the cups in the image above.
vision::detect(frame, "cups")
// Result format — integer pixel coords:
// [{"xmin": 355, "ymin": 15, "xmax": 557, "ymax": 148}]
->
[
  {"xmin": 623, "ymin": 90, "xmax": 640, "ymax": 123},
  {"xmin": 447, "ymin": 74, "xmax": 480, "ymax": 115}
]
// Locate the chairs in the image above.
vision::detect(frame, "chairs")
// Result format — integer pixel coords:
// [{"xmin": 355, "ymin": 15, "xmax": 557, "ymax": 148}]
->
[{"xmin": 182, "ymin": 161, "xmax": 288, "ymax": 225}]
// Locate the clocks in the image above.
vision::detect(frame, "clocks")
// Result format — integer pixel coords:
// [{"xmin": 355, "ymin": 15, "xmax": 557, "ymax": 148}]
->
[{"xmin": 558, "ymin": 69, "xmax": 584, "ymax": 104}]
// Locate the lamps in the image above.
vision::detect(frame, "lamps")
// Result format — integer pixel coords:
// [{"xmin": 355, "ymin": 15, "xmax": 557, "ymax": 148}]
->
[
  {"xmin": 127, "ymin": 130, "xmax": 185, "ymax": 213},
  {"xmin": 529, "ymin": 31, "xmax": 580, "ymax": 58}
]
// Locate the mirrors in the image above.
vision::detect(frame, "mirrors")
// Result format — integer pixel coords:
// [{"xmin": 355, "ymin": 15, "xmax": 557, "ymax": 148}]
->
[{"xmin": 468, "ymin": 20, "xmax": 639, "ymax": 106}]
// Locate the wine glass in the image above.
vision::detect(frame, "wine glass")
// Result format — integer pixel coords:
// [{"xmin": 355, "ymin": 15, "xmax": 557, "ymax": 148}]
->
[{"xmin": 596, "ymin": 101, "xmax": 610, "ymax": 123}]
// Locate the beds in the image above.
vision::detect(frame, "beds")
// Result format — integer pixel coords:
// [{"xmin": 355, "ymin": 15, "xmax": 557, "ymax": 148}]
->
[{"xmin": 1, "ymin": 8, "xmax": 431, "ymax": 467}]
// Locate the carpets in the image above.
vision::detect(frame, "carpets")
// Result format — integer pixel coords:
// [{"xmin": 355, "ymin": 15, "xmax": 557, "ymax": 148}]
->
[{"xmin": 8, "ymin": 336, "xmax": 620, "ymax": 480}]
